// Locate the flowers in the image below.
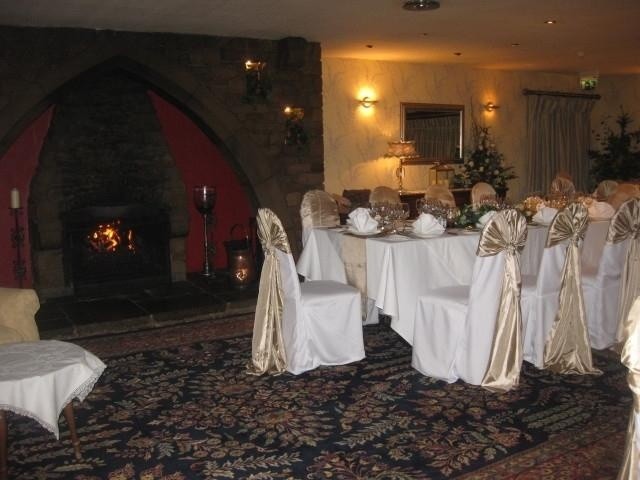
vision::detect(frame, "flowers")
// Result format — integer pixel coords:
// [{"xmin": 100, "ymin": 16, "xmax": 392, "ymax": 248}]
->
[{"xmin": 454, "ymin": 126, "xmax": 514, "ymax": 186}]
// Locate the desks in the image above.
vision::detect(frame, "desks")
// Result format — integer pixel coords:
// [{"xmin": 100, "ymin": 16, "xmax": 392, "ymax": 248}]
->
[
  {"xmin": 253, "ymin": 207, "xmax": 369, "ymax": 376},
  {"xmin": 295, "ymin": 204, "xmax": 637, "ymax": 348},
  {"xmin": 0, "ymin": 340, "xmax": 107, "ymax": 480}
]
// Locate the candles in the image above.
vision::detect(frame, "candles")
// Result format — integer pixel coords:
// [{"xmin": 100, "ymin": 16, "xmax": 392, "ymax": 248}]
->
[{"xmin": 10, "ymin": 189, "xmax": 20, "ymax": 210}]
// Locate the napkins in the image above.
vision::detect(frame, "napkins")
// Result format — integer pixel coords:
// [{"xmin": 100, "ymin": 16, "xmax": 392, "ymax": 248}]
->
[
  {"xmin": 475, "ymin": 210, "xmax": 497, "ymax": 229},
  {"xmin": 345, "ymin": 207, "xmax": 383, "ymax": 237},
  {"xmin": 533, "ymin": 205, "xmax": 561, "ymax": 226},
  {"xmin": 410, "ymin": 213, "xmax": 447, "ymax": 238},
  {"xmin": 585, "ymin": 200, "xmax": 615, "ymax": 221}
]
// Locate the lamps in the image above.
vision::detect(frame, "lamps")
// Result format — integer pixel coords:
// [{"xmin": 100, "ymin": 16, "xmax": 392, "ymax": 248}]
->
[
  {"xmin": 358, "ymin": 96, "xmax": 379, "ymax": 109},
  {"xmin": 385, "ymin": 140, "xmax": 421, "ymax": 193},
  {"xmin": 228, "ymin": 222, "xmax": 256, "ymax": 290},
  {"xmin": 244, "ymin": 56, "xmax": 267, "ymax": 98},
  {"xmin": 193, "ymin": 186, "xmax": 217, "ymax": 278},
  {"xmin": 283, "ymin": 106, "xmax": 308, "ymax": 146},
  {"xmin": 482, "ymin": 102, "xmax": 501, "ymax": 113}
]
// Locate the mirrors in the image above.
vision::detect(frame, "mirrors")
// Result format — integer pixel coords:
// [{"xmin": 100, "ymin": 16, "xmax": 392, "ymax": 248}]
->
[{"xmin": 399, "ymin": 101, "xmax": 466, "ymax": 165}]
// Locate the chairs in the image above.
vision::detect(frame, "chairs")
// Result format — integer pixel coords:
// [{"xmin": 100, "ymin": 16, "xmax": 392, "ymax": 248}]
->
[
  {"xmin": 509, "ymin": 198, "xmax": 607, "ymax": 379},
  {"xmin": 409, "ymin": 206, "xmax": 530, "ymax": 399},
  {"xmin": 422, "ymin": 184, "xmax": 457, "ymax": 212},
  {"xmin": 591, "ymin": 179, "xmax": 615, "ymax": 198},
  {"xmin": 605, "ymin": 183, "xmax": 637, "ymax": 209},
  {"xmin": 469, "ymin": 181, "xmax": 499, "ymax": 209},
  {"xmin": 0, "ymin": 288, "xmax": 41, "ymax": 342},
  {"xmin": 574, "ymin": 194, "xmax": 637, "ymax": 354},
  {"xmin": 369, "ymin": 184, "xmax": 403, "ymax": 217},
  {"xmin": 295, "ymin": 188, "xmax": 344, "ymax": 248},
  {"xmin": 548, "ymin": 179, "xmax": 578, "ymax": 200},
  {"xmin": 615, "ymin": 295, "xmax": 639, "ymax": 476}
]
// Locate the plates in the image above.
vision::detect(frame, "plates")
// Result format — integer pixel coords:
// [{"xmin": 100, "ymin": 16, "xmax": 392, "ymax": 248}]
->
[
  {"xmin": 527, "ymin": 224, "xmax": 542, "ymax": 229},
  {"xmin": 410, "ymin": 234, "xmax": 441, "ymax": 239},
  {"xmin": 337, "ymin": 224, "xmax": 393, "ymax": 238}
]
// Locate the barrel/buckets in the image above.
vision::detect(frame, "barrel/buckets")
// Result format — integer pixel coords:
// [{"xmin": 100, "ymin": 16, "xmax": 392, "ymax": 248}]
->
[{"xmin": 224, "ymin": 221, "xmax": 257, "ymax": 286}]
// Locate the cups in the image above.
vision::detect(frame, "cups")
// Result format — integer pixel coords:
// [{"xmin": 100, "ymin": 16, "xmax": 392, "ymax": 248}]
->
[{"xmin": 446, "ymin": 208, "xmax": 461, "ymax": 226}]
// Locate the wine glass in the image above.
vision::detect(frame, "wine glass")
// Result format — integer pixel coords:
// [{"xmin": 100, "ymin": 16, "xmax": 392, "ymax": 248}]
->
[{"xmin": 368, "ymin": 197, "xmax": 448, "ymax": 236}]
